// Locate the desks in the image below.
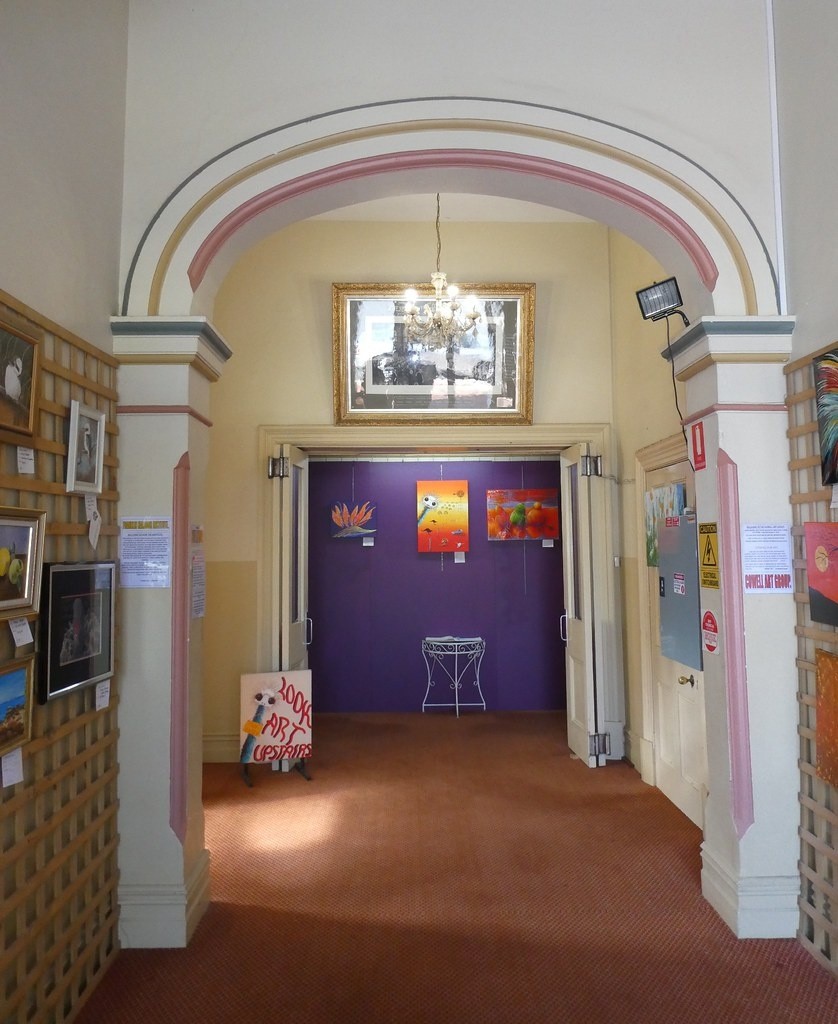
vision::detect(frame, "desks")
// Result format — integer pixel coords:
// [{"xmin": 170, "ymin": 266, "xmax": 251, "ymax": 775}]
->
[{"xmin": 420, "ymin": 639, "xmax": 486, "ymax": 719}]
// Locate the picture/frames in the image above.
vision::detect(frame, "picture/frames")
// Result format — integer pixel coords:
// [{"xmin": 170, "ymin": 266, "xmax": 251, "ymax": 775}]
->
[
  {"xmin": 0, "ymin": 321, "xmax": 40, "ymax": 437},
  {"xmin": 331, "ymin": 282, "xmax": 536, "ymax": 425},
  {"xmin": 0, "ymin": 505, "xmax": 47, "ymax": 621},
  {"xmin": 66, "ymin": 399, "xmax": 106, "ymax": 495},
  {"xmin": 0, "ymin": 653, "xmax": 36, "ymax": 758},
  {"xmin": 36, "ymin": 561, "xmax": 115, "ymax": 707}
]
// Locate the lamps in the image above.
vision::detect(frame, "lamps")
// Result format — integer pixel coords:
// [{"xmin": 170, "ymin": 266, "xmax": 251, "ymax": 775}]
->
[
  {"xmin": 635, "ymin": 276, "xmax": 690, "ymax": 328},
  {"xmin": 403, "ymin": 193, "xmax": 482, "ymax": 353}
]
